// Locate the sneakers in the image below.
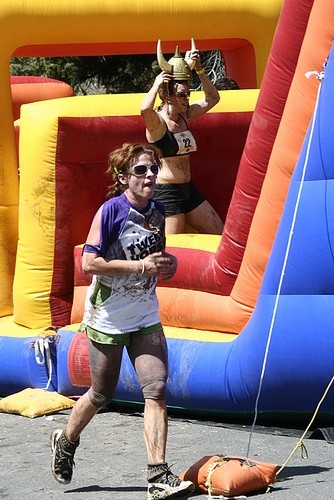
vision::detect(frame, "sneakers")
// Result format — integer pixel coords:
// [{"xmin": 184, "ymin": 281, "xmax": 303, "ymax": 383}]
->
[
  {"xmin": 147, "ymin": 463, "xmax": 194, "ymax": 500},
  {"xmin": 51, "ymin": 428, "xmax": 80, "ymax": 484}
]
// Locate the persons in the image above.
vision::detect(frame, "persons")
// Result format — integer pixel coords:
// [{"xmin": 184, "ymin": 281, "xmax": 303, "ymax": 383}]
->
[
  {"xmin": 140, "ymin": 50, "xmax": 225, "ymax": 235},
  {"xmin": 49, "ymin": 143, "xmax": 196, "ymax": 500}
]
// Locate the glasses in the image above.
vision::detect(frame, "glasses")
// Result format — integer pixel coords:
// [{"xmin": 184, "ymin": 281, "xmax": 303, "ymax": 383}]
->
[
  {"xmin": 173, "ymin": 91, "xmax": 190, "ymax": 97},
  {"xmin": 122, "ymin": 164, "xmax": 159, "ymax": 176}
]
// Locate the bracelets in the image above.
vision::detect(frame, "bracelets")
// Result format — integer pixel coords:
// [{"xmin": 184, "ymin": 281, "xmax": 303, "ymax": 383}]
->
[
  {"xmin": 196, "ymin": 68, "xmax": 205, "ymax": 75},
  {"xmin": 139, "ymin": 260, "xmax": 145, "ymax": 275}
]
